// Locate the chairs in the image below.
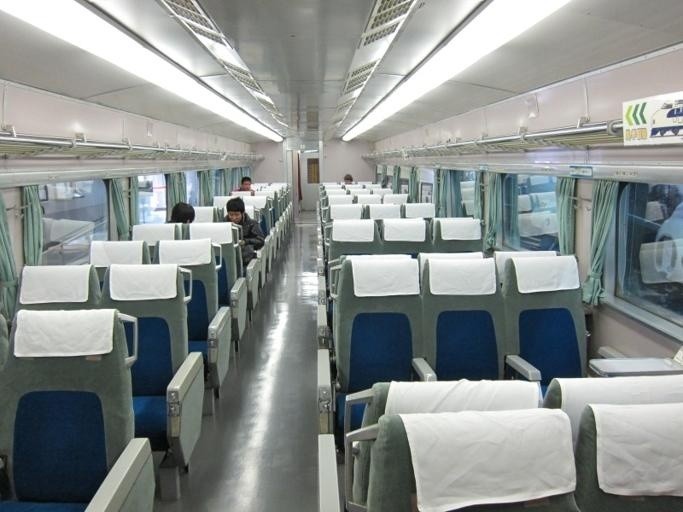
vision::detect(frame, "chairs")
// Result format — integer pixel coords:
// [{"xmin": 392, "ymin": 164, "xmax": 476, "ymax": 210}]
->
[
  {"xmin": 41, "ymin": 218, "xmax": 95, "ymax": 264},
  {"xmin": 195, "ymin": 182, "xmax": 292, "ymax": 325},
  {"xmin": 317, "ymin": 182, "xmax": 682, "ymax": 512},
  {"xmin": 2, "ymin": 263, "xmax": 205, "ymax": 511},
  {"xmin": 87, "ymin": 221, "xmax": 248, "ymax": 415}
]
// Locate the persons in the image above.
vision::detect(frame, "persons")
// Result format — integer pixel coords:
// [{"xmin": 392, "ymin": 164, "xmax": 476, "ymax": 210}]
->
[
  {"xmin": 163, "ymin": 203, "xmax": 195, "ymax": 224},
  {"xmin": 216, "ymin": 197, "xmax": 265, "ymax": 267},
  {"xmin": 229, "ymin": 176, "xmax": 255, "ymax": 196},
  {"xmin": 341, "ymin": 174, "xmax": 354, "ymax": 190}
]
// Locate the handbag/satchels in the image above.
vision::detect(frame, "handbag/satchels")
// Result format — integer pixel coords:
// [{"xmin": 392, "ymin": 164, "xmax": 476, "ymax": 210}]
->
[{"xmin": 241, "ymin": 244, "xmax": 257, "ymax": 266}]
